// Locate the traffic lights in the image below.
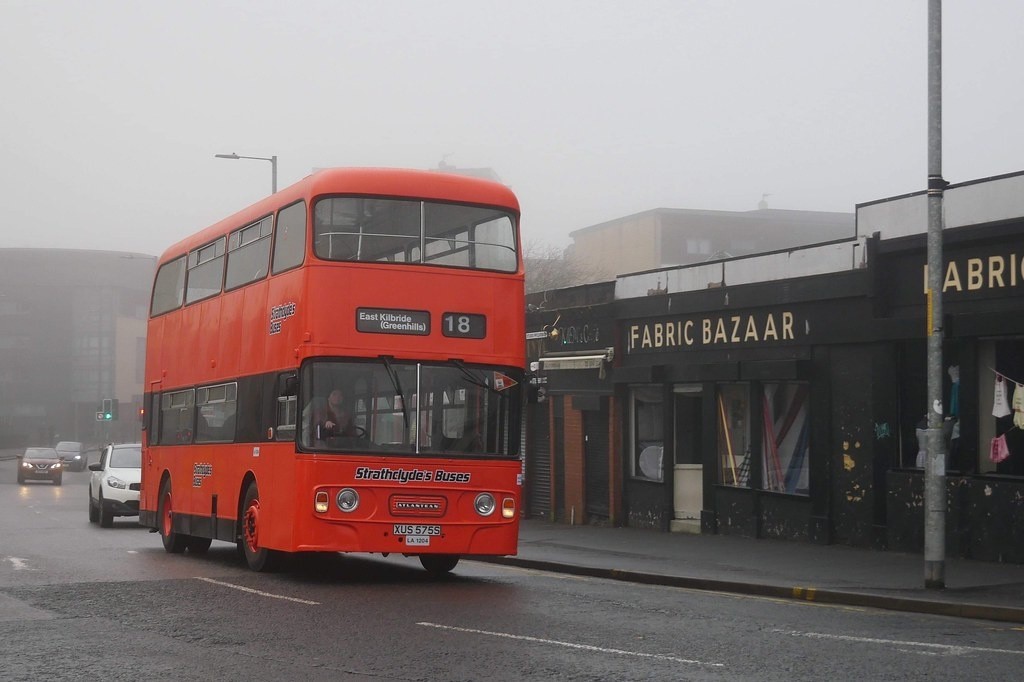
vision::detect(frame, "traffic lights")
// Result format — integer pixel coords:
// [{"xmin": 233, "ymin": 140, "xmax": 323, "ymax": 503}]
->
[{"xmin": 96, "ymin": 399, "xmax": 112, "ymax": 422}]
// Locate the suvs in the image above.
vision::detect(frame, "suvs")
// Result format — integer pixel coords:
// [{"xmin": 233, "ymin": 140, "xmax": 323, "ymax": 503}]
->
[{"xmin": 89, "ymin": 442, "xmax": 141, "ymax": 528}]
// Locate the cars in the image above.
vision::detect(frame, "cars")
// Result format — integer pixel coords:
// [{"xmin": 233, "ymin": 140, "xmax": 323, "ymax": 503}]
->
[
  {"xmin": 55, "ymin": 441, "xmax": 89, "ymax": 472},
  {"xmin": 16, "ymin": 447, "xmax": 66, "ymax": 486}
]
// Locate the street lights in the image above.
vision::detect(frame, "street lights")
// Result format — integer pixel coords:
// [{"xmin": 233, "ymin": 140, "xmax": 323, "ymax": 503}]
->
[{"xmin": 215, "ymin": 154, "xmax": 278, "ymax": 195}]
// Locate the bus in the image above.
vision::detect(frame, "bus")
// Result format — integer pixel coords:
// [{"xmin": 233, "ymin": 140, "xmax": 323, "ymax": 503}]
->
[{"xmin": 140, "ymin": 165, "xmax": 540, "ymax": 577}]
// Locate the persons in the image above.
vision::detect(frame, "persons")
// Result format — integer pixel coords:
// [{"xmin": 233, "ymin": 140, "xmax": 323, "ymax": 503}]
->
[{"xmin": 301, "ymin": 389, "xmax": 355, "ymax": 448}]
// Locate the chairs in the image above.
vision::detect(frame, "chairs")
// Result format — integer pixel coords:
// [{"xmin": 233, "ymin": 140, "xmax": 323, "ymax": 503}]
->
[{"xmin": 219, "ymin": 406, "xmax": 235, "ymax": 441}]
